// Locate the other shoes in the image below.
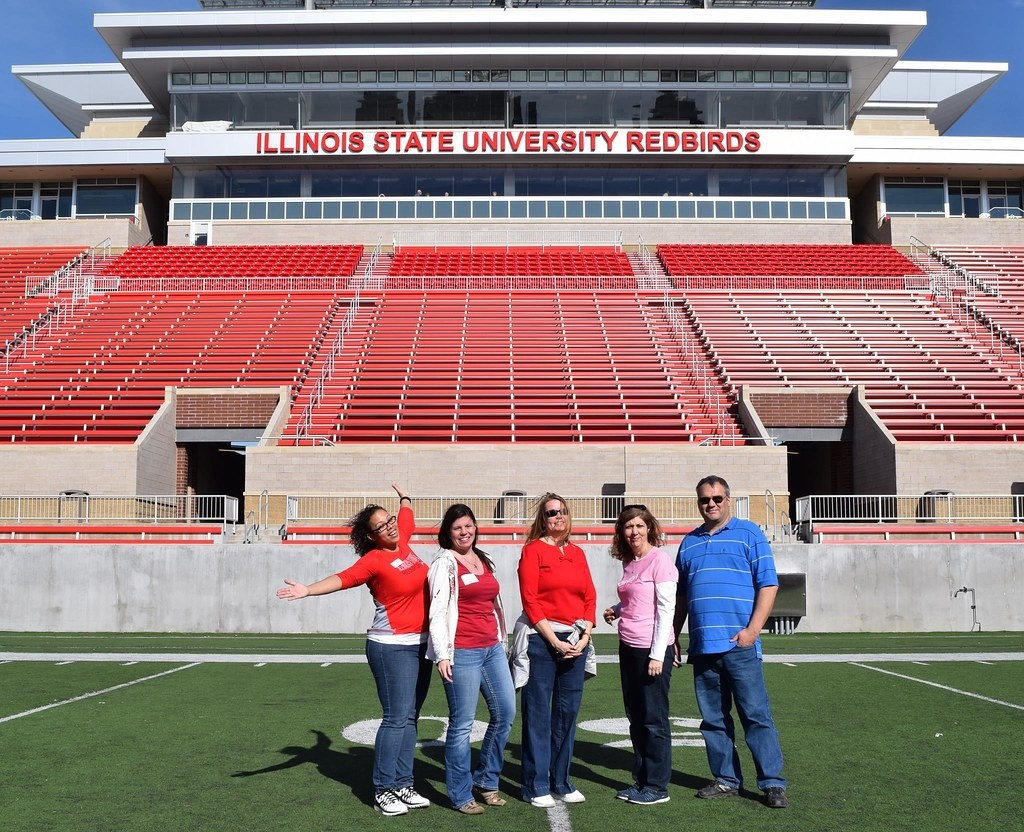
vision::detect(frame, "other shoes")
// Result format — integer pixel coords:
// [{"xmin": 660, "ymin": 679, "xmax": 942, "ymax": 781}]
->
[
  {"xmin": 472, "ymin": 786, "xmax": 506, "ymax": 806},
  {"xmin": 459, "ymin": 801, "xmax": 485, "ymax": 814}
]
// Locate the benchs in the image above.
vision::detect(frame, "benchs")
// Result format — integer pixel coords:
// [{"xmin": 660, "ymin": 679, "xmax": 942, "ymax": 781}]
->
[{"xmin": 0, "ymin": 244, "xmax": 1024, "ymax": 447}]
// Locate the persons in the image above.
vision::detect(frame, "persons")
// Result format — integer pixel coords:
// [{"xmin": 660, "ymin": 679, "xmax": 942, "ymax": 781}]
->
[
  {"xmin": 603, "ymin": 504, "xmax": 679, "ymax": 805},
  {"xmin": 674, "ymin": 475, "xmax": 786, "ymax": 808},
  {"xmin": 275, "ymin": 483, "xmax": 431, "ymax": 816},
  {"xmin": 427, "ymin": 503, "xmax": 516, "ymax": 814},
  {"xmin": 510, "ymin": 491, "xmax": 597, "ymax": 807}
]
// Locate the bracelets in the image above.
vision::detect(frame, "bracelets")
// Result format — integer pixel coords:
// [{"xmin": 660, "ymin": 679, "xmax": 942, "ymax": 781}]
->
[
  {"xmin": 400, "ymin": 496, "xmax": 412, "ymax": 504},
  {"xmin": 582, "ymin": 632, "xmax": 591, "ymax": 643}
]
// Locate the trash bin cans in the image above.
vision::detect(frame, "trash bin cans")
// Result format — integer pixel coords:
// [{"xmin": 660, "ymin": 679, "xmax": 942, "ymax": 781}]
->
[
  {"xmin": 924, "ymin": 490, "xmax": 956, "ymax": 524},
  {"xmin": 58, "ymin": 490, "xmax": 90, "ymax": 523},
  {"xmin": 502, "ymin": 490, "xmax": 527, "ymax": 524}
]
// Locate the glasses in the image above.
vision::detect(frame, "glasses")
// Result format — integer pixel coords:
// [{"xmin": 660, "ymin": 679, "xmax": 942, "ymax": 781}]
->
[
  {"xmin": 697, "ymin": 495, "xmax": 728, "ymax": 505},
  {"xmin": 370, "ymin": 516, "xmax": 396, "ymax": 534},
  {"xmin": 542, "ymin": 507, "xmax": 569, "ymax": 517},
  {"xmin": 621, "ymin": 505, "xmax": 647, "ymax": 513}
]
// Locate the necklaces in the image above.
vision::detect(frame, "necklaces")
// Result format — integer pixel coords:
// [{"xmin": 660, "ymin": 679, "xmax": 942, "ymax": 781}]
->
[{"xmin": 453, "ymin": 549, "xmax": 479, "ymax": 570}]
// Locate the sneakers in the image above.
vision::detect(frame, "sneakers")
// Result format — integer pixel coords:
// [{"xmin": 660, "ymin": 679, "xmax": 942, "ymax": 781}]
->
[
  {"xmin": 523, "ymin": 793, "xmax": 555, "ymax": 808},
  {"xmin": 697, "ymin": 779, "xmax": 744, "ymax": 799},
  {"xmin": 393, "ymin": 786, "xmax": 430, "ymax": 808},
  {"xmin": 763, "ymin": 786, "xmax": 788, "ymax": 807},
  {"xmin": 374, "ymin": 791, "xmax": 408, "ymax": 816},
  {"xmin": 550, "ymin": 789, "xmax": 585, "ymax": 803},
  {"xmin": 628, "ymin": 787, "xmax": 670, "ymax": 805},
  {"xmin": 617, "ymin": 783, "xmax": 644, "ymax": 801}
]
